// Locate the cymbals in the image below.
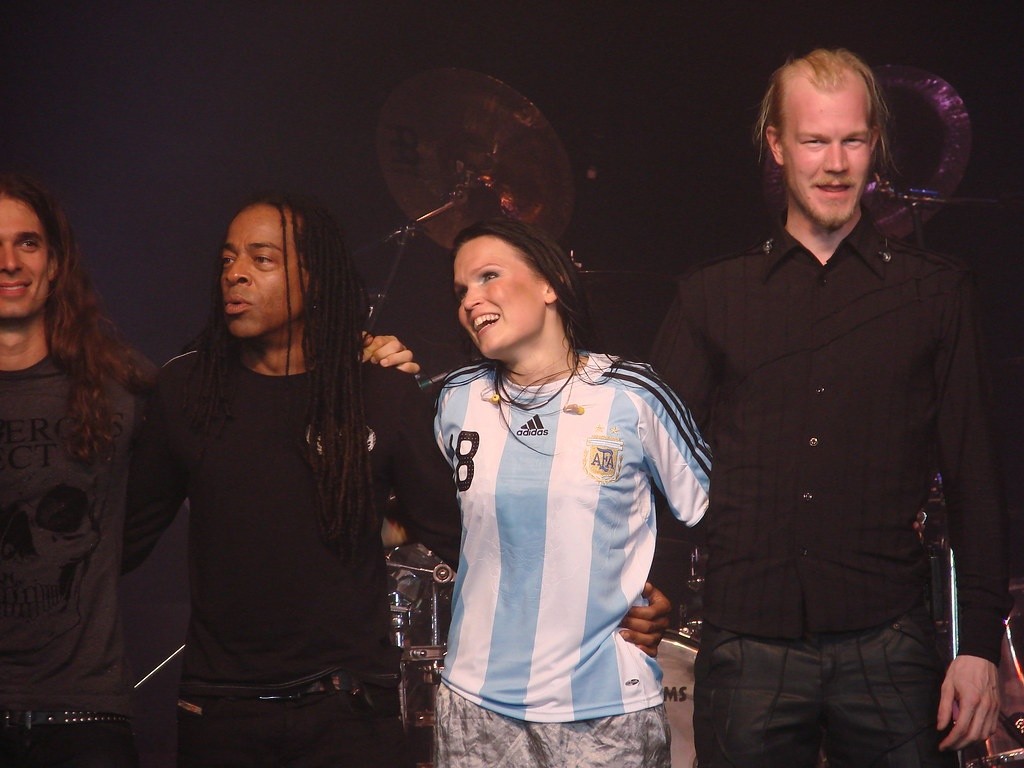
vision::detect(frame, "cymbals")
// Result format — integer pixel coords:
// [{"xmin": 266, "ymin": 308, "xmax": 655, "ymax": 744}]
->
[
  {"xmin": 757, "ymin": 64, "xmax": 970, "ymax": 243},
  {"xmin": 372, "ymin": 66, "xmax": 574, "ymax": 255}
]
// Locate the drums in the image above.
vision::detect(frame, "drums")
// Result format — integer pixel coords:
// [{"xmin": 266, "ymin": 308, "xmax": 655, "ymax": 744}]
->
[
  {"xmin": 647, "ymin": 536, "xmax": 707, "ymax": 648},
  {"xmin": 396, "ymin": 644, "xmax": 446, "ymax": 732},
  {"xmin": 954, "ymin": 576, "xmax": 1024, "ymax": 768},
  {"xmin": 387, "ymin": 604, "xmax": 434, "ymax": 651}
]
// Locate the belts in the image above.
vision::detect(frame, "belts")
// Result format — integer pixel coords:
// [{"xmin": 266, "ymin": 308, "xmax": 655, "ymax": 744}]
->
[
  {"xmin": 0, "ymin": 712, "xmax": 131, "ymax": 730},
  {"xmin": 257, "ymin": 675, "xmax": 349, "ymax": 700}
]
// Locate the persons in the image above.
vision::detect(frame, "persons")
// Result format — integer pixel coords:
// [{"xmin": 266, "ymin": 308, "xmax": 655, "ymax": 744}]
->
[
  {"xmin": 640, "ymin": 44, "xmax": 1014, "ymax": 768},
  {"xmin": 118, "ymin": 187, "xmax": 672, "ymax": 768},
  {"xmin": 0, "ymin": 174, "xmax": 420, "ymax": 768},
  {"xmin": 375, "ymin": 215, "xmax": 927, "ymax": 768}
]
